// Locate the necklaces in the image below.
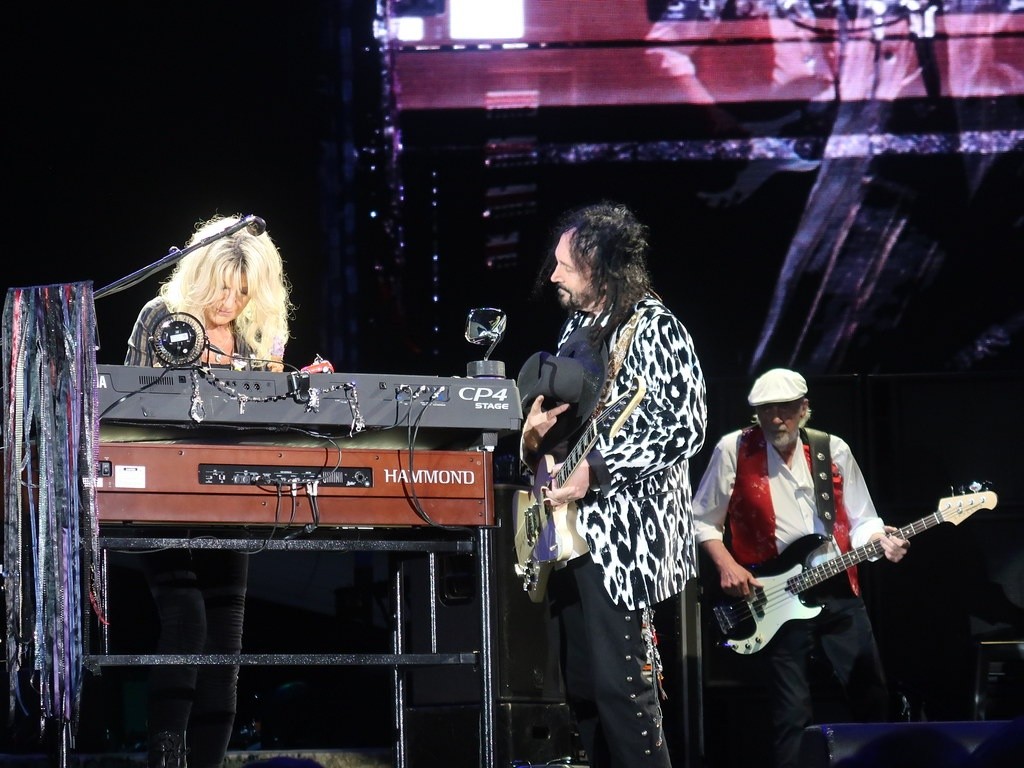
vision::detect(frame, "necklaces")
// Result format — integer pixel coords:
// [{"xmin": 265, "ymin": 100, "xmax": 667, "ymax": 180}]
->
[{"xmin": 204, "ymin": 329, "xmax": 232, "ymax": 363}]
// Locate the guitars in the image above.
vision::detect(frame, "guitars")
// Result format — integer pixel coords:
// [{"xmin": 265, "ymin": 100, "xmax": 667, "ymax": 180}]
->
[
  {"xmin": 708, "ymin": 481, "xmax": 998, "ymax": 657},
  {"xmin": 510, "ymin": 376, "xmax": 647, "ymax": 604}
]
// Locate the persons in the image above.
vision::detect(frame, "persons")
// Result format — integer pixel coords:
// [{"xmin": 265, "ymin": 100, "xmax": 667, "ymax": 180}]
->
[
  {"xmin": 122, "ymin": 216, "xmax": 292, "ymax": 767},
  {"xmin": 693, "ymin": 365, "xmax": 912, "ymax": 767},
  {"xmin": 514, "ymin": 201, "xmax": 710, "ymax": 768}
]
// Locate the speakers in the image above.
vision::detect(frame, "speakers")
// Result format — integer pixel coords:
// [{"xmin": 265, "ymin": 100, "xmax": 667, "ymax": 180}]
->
[
  {"xmin": 406, "ymin": 485, "xmax": 576, "ymax": 768},
  {"xmin": 968, "ymin": 636, "xmax": 1024, "ymax": 720},
  {"xmin": 796, "ymin": 720, "xmax": 1024, "ymax": 768}
]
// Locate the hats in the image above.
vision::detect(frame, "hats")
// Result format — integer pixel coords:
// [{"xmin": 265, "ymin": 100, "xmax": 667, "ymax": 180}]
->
[
  {"xmin": 517, "ymin": 326, "xmax": 609, "ymax": 465},
  {"xmin": 748, "ymin": 369, "xmax": 808, "ymax": 406}
]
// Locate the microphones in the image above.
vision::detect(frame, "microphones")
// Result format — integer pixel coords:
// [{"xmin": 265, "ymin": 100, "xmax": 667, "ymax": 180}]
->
[{"xmin": 245, "ymin": 216, "xmax": 267, "ymax": 237}]
